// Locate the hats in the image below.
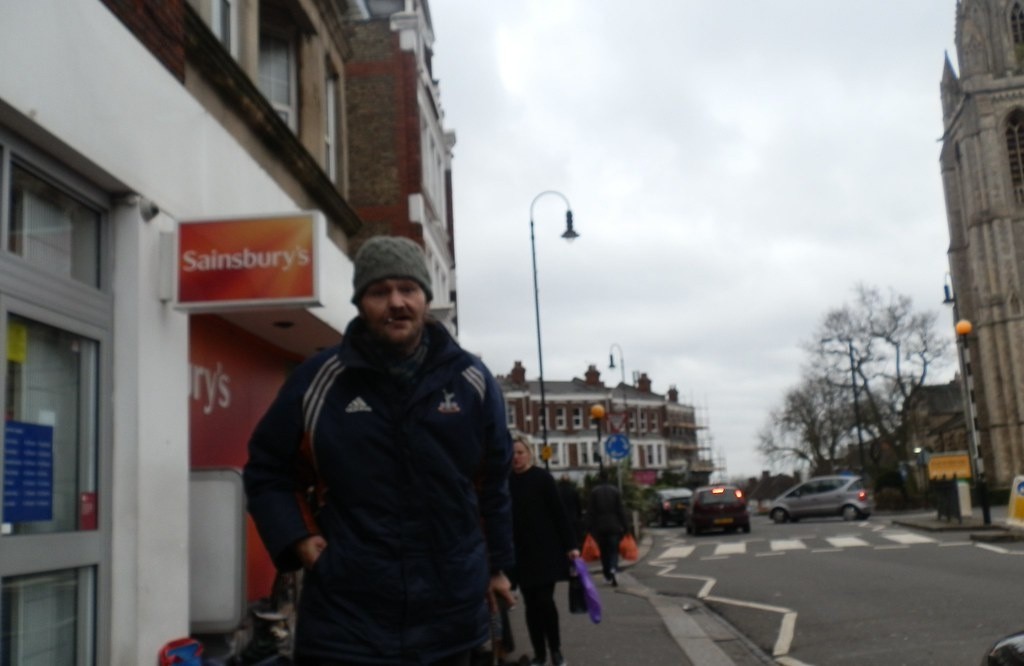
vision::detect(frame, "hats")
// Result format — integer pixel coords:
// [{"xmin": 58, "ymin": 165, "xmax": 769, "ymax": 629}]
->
[{"xmin": 352, "ymin": 236, "xmax": 434, "ymax": 302}]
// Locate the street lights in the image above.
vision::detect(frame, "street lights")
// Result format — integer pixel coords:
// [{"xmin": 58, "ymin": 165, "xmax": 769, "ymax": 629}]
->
[
  {"xmin": 591, "ymin": 404, "xmax": 605, "ymax": 481},
  {"xmin": 609, "ymin": 344, "xmax": 630, "ymax": 442},
  {"xmin": 529, "ymin": 189, "xmax": 580, "ymax": 475},
  {"xmin": 820, "ymin": 337, "xmax": 866, "ymax": 473},
  {"xmin": 956, "ymin": 320, "xmax": 991, "ymax": 525}
]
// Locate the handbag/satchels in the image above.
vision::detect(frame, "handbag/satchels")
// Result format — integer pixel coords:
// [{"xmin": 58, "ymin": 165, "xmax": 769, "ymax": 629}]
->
[
  {"xmin": 570, "ymin": 557, "xmax": 603, "ymax": 625},
  {"xmin": 620, "ymin": 533, "xmax": 637, "ymax": 560},
  {"xmin": 582, "ymin": 533, "xmax": 600, "ymax": 561}
]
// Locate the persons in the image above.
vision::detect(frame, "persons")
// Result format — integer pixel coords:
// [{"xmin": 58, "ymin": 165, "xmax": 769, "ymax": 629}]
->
[
  {"xmin": 557, "ymin": 468, "xmax": 630, "ymax": 587},
  {"xmin": 509, "ymin": 431, "xmax": 580, "ymax": 666},
  {"xmin": 243, "ymin": 235, "xmax": 517, "ymax": 665}
]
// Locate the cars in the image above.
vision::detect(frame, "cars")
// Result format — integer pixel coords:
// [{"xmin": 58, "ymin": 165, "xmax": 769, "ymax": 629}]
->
[
  {"xmin": 767, "ymin": 475, "xmax": 876, "ymax": 524},
  {"xmin": 686, "ymin": 485, "xmax": 751, "ymax": 537},
  {"xmin": 645, "ymin": 488, "xmax": 692, "ymax": 529}
]
